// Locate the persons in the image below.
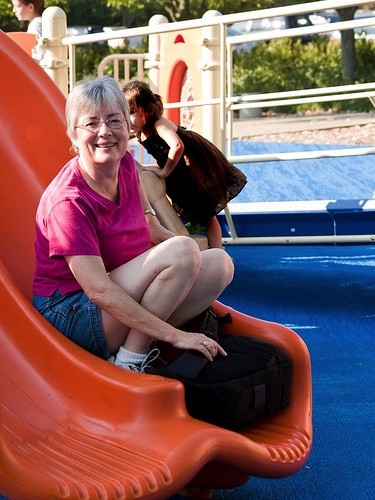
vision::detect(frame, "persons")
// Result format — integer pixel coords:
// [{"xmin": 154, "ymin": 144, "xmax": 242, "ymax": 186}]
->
[
  {"xmin": 12, "ymin": 0, "xmax": 45, "ymax": 66},
  {"xmin": 33, "ymin": 77, "xmax": 235, "ymax": 373},
  {"xmin": 120, "ymin": 80, "xmax": 247, "ymax": 264}
]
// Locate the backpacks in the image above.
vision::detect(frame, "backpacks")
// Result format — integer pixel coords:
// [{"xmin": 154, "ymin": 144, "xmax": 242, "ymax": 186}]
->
[{"xmin": 146, "ymin": 305, "xmax": 293, "ymax": 433}]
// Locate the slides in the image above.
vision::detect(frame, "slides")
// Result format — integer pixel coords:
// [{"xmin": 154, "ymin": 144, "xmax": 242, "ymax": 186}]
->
[{"xmin": 0, "ymin": 28, "xmax": 314, "ymax": 496}]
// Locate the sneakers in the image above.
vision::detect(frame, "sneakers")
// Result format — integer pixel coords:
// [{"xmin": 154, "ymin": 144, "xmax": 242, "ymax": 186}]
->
[{"xmin": 108, "ymin": 355, "xmax": 148, "ymax": 375}]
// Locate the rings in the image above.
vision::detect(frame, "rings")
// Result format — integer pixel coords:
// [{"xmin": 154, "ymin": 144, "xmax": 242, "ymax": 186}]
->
[{"xmin": 202, "ymin": 341, "xmax": 210, "ymax": 346}]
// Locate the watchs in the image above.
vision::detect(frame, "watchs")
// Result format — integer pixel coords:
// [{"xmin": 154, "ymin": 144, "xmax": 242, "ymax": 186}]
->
[{"xmin": 144, "ymin": 208, "xmax": 156, "ymax": 216}]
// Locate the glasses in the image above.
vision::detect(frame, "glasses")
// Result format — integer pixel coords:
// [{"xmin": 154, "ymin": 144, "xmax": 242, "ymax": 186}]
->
[{"xmin": 74, "ymin": 116, "xmax": 129, "ymax": 132}]
